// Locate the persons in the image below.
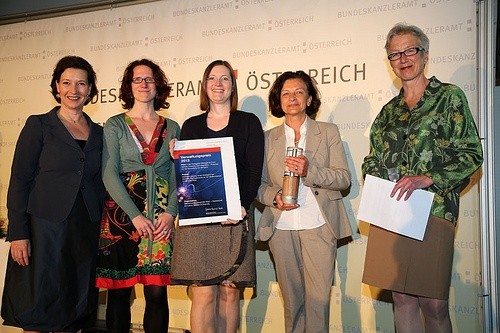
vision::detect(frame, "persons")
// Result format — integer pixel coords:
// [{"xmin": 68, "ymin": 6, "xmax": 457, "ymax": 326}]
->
[
  {"xmin": 361, "ymin": 24, "xmax": 484, "ymax": 333},
  {"xmin": 1, "ymin": 55, "xmax": 104, "ymax": 333},
  {"xmin": 96, "ymin": 59, "xmax": 181, "ymax": 333},
  {"xmin": 168, "ymin": 61, "xmax": 264, "ymax": 332},
  {"xmin": 256, "ymin": 71, "xmax": 352, "ymax": 333}
]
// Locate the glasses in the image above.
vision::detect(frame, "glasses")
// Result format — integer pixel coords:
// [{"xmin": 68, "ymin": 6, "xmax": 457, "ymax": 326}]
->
[
  {"xmin": 132, "ymin": 77, "xmax": 156, "ymax": 83},
  {"xmin": 207, "ymin": 76, "xmax": 233, "ymax": 82},
  {"xmin": 388, "ymin": 48, "xmax": 424, "ymax": 60}
]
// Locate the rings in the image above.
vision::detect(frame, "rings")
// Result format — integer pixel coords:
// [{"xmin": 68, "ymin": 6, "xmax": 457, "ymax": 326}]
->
[
  {"xmin": 164, "ymin": 231, "xmax": 167, "ymax": 234},
  {"xmin": 143, "ymin": 231, "xmax": 147, "ymax": 233},
  {"xmin": 295, "ymin": 167, "xmax": 298, "ymax": 170}
]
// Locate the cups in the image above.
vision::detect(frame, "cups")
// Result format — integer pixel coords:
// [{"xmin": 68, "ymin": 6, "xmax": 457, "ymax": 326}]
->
[{"xmin": 282, "ymin": 147, "xmax": 303, "ymax": 204}]
